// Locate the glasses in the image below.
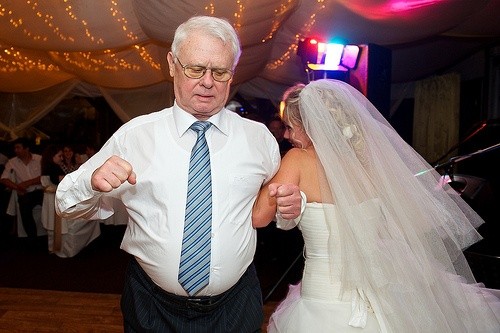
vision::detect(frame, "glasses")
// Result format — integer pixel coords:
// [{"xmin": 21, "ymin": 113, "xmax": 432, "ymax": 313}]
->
[{"xmin": 175, "ymin": 54, "xmax": 235, "ymax": 82}]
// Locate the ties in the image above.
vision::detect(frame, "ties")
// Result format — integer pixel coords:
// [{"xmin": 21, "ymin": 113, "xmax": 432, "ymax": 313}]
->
[{"xmin": 178, "ymin": 120, "xmax": 213, "ymax": 296}]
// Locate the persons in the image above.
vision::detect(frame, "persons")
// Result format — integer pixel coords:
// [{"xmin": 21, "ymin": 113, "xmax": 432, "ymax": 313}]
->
[
  {"xmin": 0, "ymin": 137, "xmax": 127, "ymax": 260},
  {"xmin": 252, "ymin": 79, "xmax": 500, "ymax": 333},
  {"xmin": 55, "ymin": 16, "xmax": 307, "ymax": 333},
  {"xmin": 268, "ymin": 116, "xmax": 295, "ymax": 160}
]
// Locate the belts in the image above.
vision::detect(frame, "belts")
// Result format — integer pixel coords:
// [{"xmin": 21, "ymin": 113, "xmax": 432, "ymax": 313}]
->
[{"xmin": 133, "ymin": 257, "xmax": 254, "ymax": 311}]
